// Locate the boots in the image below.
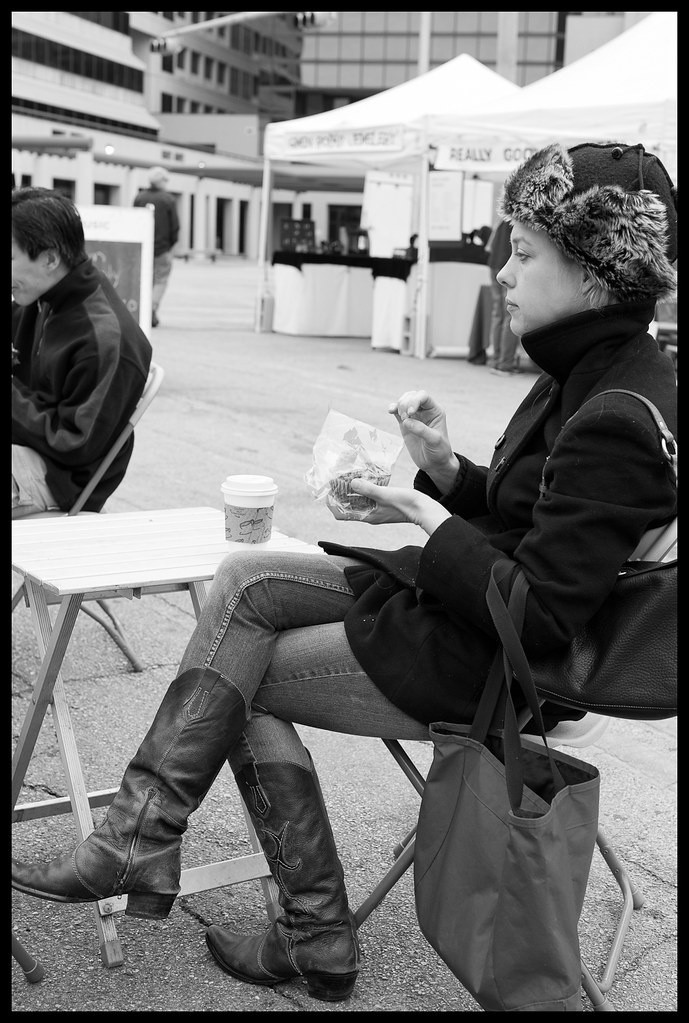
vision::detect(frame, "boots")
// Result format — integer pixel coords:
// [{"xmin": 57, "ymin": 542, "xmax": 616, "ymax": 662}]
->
[
  {"xmin": 207, "ymin": 747, "xmax": 359, "ymax": 1001},
  {"xmin": 10, "ymin": 665, "xmax": 246, "ymax": 920}
]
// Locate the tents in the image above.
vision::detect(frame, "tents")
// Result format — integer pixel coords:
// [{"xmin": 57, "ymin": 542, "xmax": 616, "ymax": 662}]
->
[{"xmin": 255, "ymin": 10, "xmax": 678, "ymax": 359}]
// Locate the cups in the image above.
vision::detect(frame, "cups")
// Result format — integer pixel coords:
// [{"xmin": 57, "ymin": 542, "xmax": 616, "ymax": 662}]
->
[{"xmin": 221, "ymin": 474, "xmax": 278, "ymax": 552}]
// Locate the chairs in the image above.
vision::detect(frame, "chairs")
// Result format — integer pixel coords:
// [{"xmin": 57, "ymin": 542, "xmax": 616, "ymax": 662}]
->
[
  {"xmin": 10, "ymin": 361, "xmax": 163, "ymax": 675},
  {"xmin": 355, "ymin": 515, "xmax": 677, "ymax": 1012}
]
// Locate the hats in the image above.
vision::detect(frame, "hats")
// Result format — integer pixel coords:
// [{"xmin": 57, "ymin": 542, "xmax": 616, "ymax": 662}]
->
[{"xmin": 502, "ymin": 138, "xmax": 680, "ymax": 305}]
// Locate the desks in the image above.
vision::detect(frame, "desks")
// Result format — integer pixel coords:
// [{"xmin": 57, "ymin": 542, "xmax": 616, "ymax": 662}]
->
[
  {"xmin": 372, "ymin": 257, "xmax": 412, "ymax": 353},
  {"xmin": 10, "ymin": 506, "xmax": 328, "ymax": 970},
  {"xmin": 272, "ymin": 252, "xmax": 373, "ymax": 338},
  {"xmin": 406, "ymin": 242, "xmax": 493, "ymax": 358}
]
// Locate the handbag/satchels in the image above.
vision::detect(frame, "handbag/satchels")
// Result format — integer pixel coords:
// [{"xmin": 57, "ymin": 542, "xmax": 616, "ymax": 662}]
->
[
  {"xmin": 415, "ymin": 562, "xmax": 599, "ymax": 1011},
  {"xmin": 519, "ymin": 389, "xmax": 678, "ymax": 721}
]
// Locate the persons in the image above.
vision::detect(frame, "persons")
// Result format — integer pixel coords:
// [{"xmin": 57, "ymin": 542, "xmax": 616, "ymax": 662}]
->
[
  {"xmin": 12, "ymin": 186, "xmax": 152, "ymax": 521},
  {"xmin": 487, "ymin": 220, "xmax": 518, "ymax": 374},
  {"xmin": 370, "ymin": 257, "xmax": 411, "ymax": 351},
  {"xmin": 134, "ymin": 166, "xmax": 180, "ymax": 328},
  {"xmin": 272, "ymin": 238, "xmax": 343, "ymax": 271},
  {"xmin": 9, "ymin": 142, "xmax": 680, "ymax": 1002}
]
